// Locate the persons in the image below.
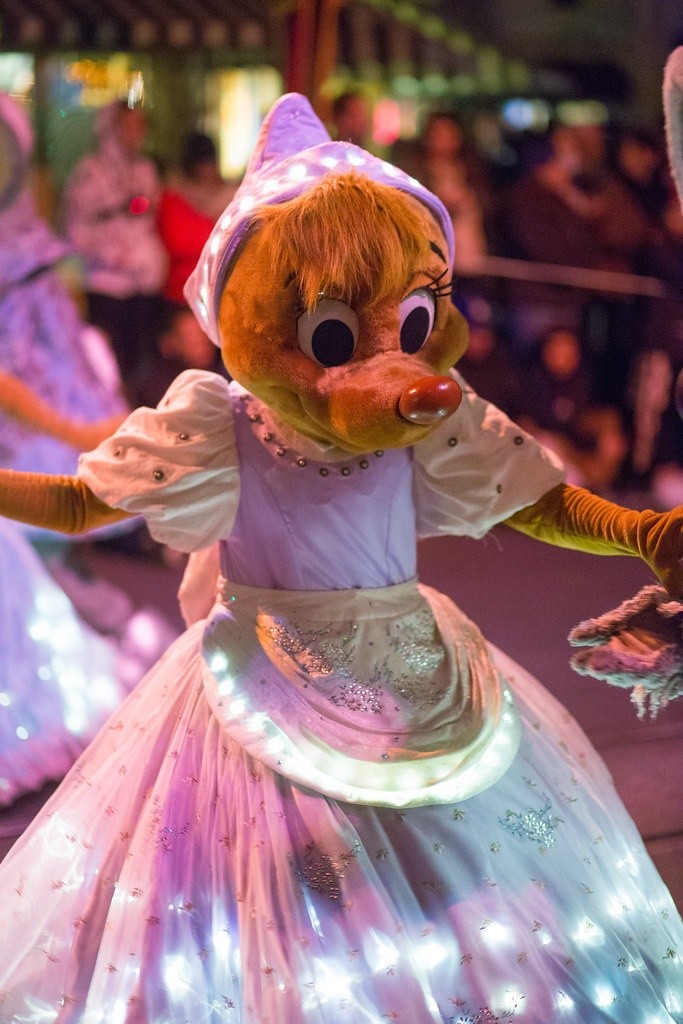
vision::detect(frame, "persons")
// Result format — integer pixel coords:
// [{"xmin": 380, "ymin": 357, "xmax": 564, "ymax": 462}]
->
[
  {"xmin": 330, "ymin": 85, "xmax": 683, "ymax": 492},
  {"xmin": 58, "ymin": 96, "xmax": 242, "ymax": 381}
]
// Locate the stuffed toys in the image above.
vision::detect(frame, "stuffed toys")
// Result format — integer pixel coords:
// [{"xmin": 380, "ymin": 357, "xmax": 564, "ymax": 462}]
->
[
  {"xmin": 0, "ymin": 117, "xmax": 178, "ymax": 840},
  {"xmin": 0, "ymin": 93, "xmax": 683, "ymax": 1024}
]
